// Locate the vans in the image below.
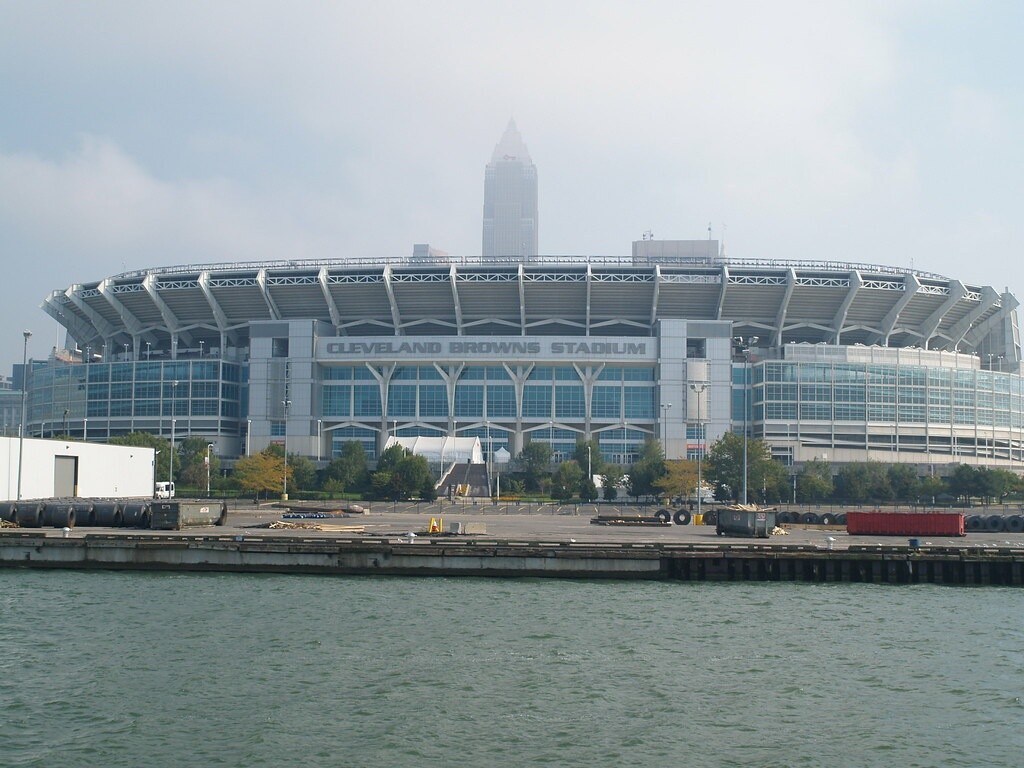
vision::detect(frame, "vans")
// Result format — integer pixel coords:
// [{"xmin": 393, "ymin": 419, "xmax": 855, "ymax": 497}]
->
[{"xmin": 156, "ymin": 481, "xmax": 175, "ymax": 499}]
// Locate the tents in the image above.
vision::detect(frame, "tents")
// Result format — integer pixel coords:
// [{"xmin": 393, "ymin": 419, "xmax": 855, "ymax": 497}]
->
[{"xmin": 384, "ymin": 436, "xmax": 486, "ymax": 479}]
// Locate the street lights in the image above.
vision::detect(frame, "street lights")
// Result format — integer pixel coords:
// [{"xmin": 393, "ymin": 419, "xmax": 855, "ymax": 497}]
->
[
  {"xmin": 924, "ymin": 450, "xmax": 935, "ymax": 505},
  {"xmin": 490, "ymin": 435, "xmax": 494, "ymax": 481},
  {"xmin": 279, "ymin": 399, "xmax": 294, "ymax": 494},
  {"xmin": 484, "ymin": 420, "xmax": 490, "ymax": 463},
  {"xmin": 658, "ymin": 402, "xmax": 672, "ymax": 480},
  {"xmin": 101, "ymin": 344, "xmax": 108, "ymax": 416},
  {"xmin": 988, "ymin": 353, "xmax": 993, "ymax": 371},
  {"xmin": 787, "ymin": 423, "xmax": 790, "ymax": 467},
  {"xmin": 84, "ymin": 346, "xmax": 93, "ymax": 418},
  {"xmin": 247, "ymin": 420, "xmax": 251, "ymax": 457},
  {"xmin": 18, "ymin": 424, "xmax": 22, "ymax": 437},
  {"xmin": 62, "ymin": 409, "xmax": 71, "ymax": 439},
  {"xmin": 624, "ymin": 420, "xmax": 628, "ymax": 464},
  {"xmin": 83, "ymin": 418, "xmax": 88, "ymax": 441},
  {"xmin": 168, "ymin": 419, "xmax": 177, "ymax": 500},
  {"xmin": 689, "ymin": 384, "xmax": 708, "ymax": 516},
  {"xmin": 392, "ymin": 419, "xmax": 398, "ymax": 445},
  {"xmin": 587, "ymin": 447, "xmax": 593, "ymax": 503},
  {"xmin": 731, "ymin": 335, "xmax": 762, "ymax": 505},
  {"xmin": 144, "ymin": 342, "xmax": 151, "ymax": 416},
  {"xmin": 452, "ymin": 420, "xmax": 458, "ymax": 461},
  {"xmin": 40, "ymin": 422, "xmax": 47, "ymax": 439},
  {"xmin": 16, "ymin": 329, "xmax": 34, "ymax": 501},
  {"xmin": 207, "ymin": 443, "xmax": 214, "ymax": 498},
  {"xmin": 196, "ymin": 340, "xmax": 204, "ymax": 415},
  {"xmin": 317, "ymin": 420, "xmax": 322, "ymax": 461},
  {"xmin": 124, "ymin": 343, "xmax": 130, "ymax": 417},
  {"xmin": 997, "ymin": 355, "xmax": 1004, "ymax": 372},
  {"xmin": 548, "ymin": 421, "xmax": 554, "ymax": 451},
  {"xmin": 700, "ymin": 422, "xmax": 705, "ymax": 460}
]
